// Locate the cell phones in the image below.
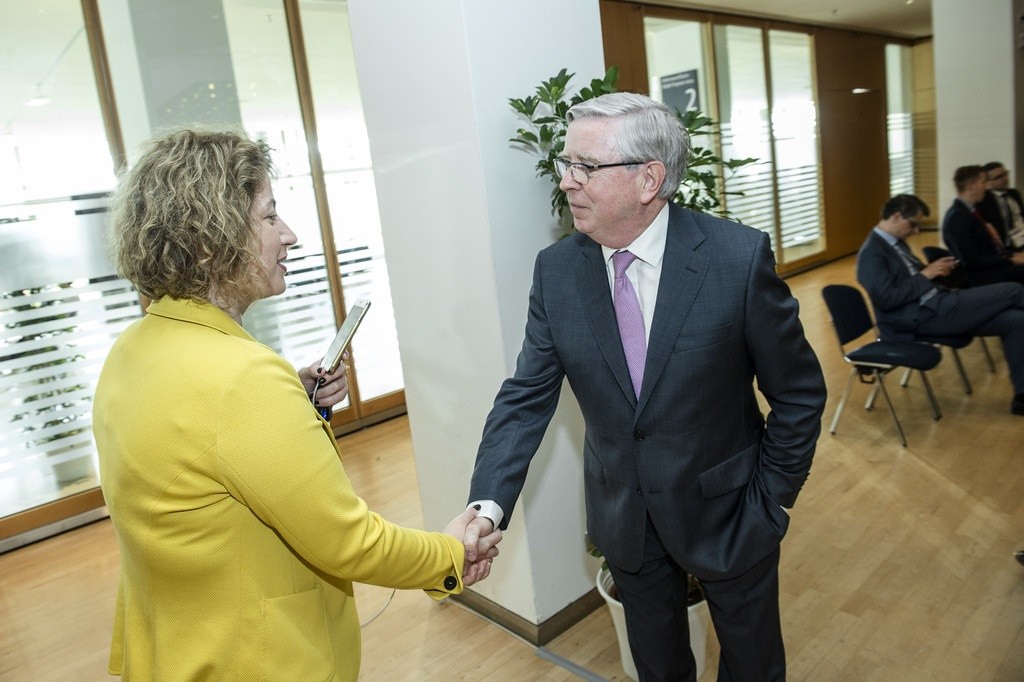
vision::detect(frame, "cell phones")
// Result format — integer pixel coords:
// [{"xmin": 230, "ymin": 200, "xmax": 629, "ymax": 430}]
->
[
  {"xmin": 318, "ymin": 298, "xmax": 371, "ymax": 374},
  {"xmin": 946, "ymin": 259, "xmax": 960, "ymax": 265}
]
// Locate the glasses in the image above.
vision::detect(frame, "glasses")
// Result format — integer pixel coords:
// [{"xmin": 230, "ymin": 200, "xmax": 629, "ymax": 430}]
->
[
  {"xmin": 553, "ymin": 157, "xmax": 649, "ymax": 185},
  {"xmin": 906, "ymin": 218, "xmax": 918, "ymax": 229}
]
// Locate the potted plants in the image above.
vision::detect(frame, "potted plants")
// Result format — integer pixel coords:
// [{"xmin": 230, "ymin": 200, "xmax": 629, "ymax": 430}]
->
[
  {"xmin": 0, "ymin": 216, "xmax": 100, "ymax": 494},
  {"xmin": 509, "ymin": 64, "xmax": 761, "ymax": 681}
]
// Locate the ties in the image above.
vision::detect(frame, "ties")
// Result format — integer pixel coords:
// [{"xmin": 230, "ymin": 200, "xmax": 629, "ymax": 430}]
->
[{"xmin": 612, "ymin": 250, "xmax": 647, "ymax": 402}]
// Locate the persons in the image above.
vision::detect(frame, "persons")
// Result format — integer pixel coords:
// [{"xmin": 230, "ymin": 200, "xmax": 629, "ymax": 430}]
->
[
  {"xmin": 462, "ymin": 92, "xmax": 830, "ymax": 682},
  {"xmin": 89, "ymin": 129, "xmax": 503, "ymax": 682},
  {"xmin": 943, "ymin": 166, "xmax": 1024, "ymax": 286},
  {"xmin": 975, "ymin": 161, "xmax": 1024, "ymax": 254},
  {"xmin": 856, "ymin": 195, "xmax": 1024, "ymax": 416}
]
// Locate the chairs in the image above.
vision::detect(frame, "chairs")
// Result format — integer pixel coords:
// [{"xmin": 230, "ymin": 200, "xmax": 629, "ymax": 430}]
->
[
  {"xmin": 821, "ymin": 284, "xmax": 945, "ymax": 448},
  {"xmin": 866, "ymin": 245, "xmax": 997, "ymax": 415}
]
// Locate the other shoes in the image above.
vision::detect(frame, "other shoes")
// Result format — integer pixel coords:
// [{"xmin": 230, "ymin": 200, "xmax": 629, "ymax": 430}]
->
[
  {"xmin": 1014, "ymin": 550, "xmax": 1024, "ymax": 565},
  {"xmin": 1010, "ymin": 399, "xmax": 1024, "ymax": 415}
]
